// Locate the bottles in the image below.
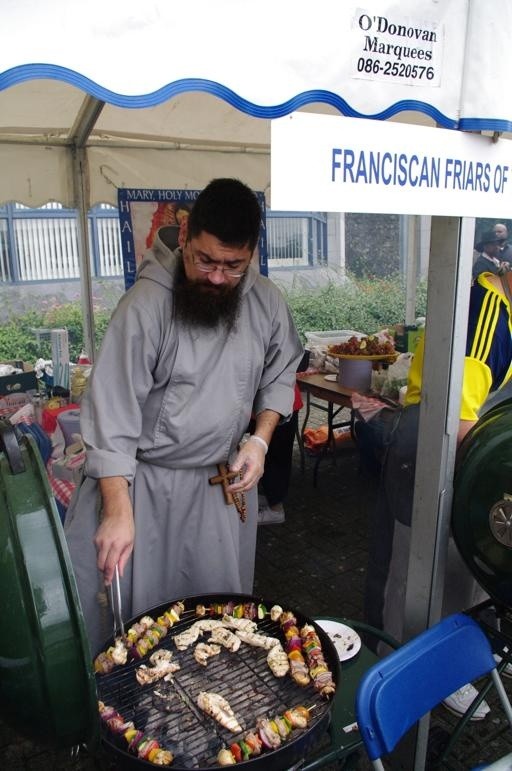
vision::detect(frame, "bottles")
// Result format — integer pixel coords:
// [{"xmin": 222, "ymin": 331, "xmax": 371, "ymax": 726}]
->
[
  {"xmin": 70, "ymin": 366, "xmax": 86, "ymax": 401},
  {"xmin": 31, "ymin": 392, "xmax": 47, "ymax": 422},
  {"xmin": 79, "ymin": 355, "xmax": 90, "ymax": 364}
]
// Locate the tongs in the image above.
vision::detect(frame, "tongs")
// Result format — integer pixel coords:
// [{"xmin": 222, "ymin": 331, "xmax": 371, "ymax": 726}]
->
[{"xmin": 106, "ymin": 563, "xmax": 129, "ymax": 647}]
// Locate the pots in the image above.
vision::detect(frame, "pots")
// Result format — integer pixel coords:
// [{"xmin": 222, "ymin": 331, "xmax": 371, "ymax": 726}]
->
[{"xmin": 90, "ymin": 588, "xmax": 344, "ymax": 770}]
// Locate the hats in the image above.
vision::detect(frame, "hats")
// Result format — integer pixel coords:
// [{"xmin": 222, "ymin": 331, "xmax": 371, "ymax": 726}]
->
[{"xmin": 476, "ymin": 231, "xmax": 505, "ymax": 252}]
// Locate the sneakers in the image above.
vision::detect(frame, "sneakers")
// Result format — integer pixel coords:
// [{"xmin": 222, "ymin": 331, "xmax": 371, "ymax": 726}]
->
[{"xmin": 257, "ymin": 504, "xmax": 286, "ymax": 525}]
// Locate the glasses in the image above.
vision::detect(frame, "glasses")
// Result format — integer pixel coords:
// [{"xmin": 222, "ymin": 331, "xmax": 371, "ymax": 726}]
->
[{"xmin": 189, "ymin": 240, "xmax": 250, "ymax": 277}]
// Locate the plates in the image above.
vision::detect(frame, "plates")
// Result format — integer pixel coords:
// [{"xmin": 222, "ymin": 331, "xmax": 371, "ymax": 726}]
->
[
  {"xmin": 324, "ymin": 374, "xmax": 338, "ymax": 382},
  {"xmin": 313, "ymin": 619, "xmax": 365, "ymax": 664}
]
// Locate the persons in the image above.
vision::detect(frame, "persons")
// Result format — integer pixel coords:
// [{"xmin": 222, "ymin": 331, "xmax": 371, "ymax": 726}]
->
[
  {"xmin": 61, "ymin": 175, "xmax": 309, "ymax": 669},
  {"xmin": 363, "ymin": 264, "xmax": 512, "ymax": 722},
  {"xmin": 492, "ymin": 223, "xmax": 512, "ymax": 263},
  {"xmin": 471, "ymin": 231, "xmax": 512, "ymax": 285},
  {"xmin": 250, "ymin": 376, "xmax": 304, "ymax": 528}
]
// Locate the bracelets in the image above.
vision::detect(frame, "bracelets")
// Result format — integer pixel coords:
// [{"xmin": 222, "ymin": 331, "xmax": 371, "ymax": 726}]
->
[{"xmin": 250, "ymin": 435, "xmax": 271, "ymax": 453}]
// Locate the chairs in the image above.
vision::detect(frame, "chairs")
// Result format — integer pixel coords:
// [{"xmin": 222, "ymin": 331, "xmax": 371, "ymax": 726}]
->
[{"xmin": 355, "ymin": 614, "xmax": 511, "ymax": 771}]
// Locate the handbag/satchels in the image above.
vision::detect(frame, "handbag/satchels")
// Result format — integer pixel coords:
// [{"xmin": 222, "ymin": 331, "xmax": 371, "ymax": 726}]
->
[{"xmin": 386, "ymin": 403, "xmax": 419, "ymax": 526}]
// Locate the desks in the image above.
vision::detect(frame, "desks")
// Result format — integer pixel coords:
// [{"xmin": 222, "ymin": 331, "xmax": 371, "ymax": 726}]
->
[{"xmin": 299, "ymin": 370, "xmax": 376, "ymax": 498}]
